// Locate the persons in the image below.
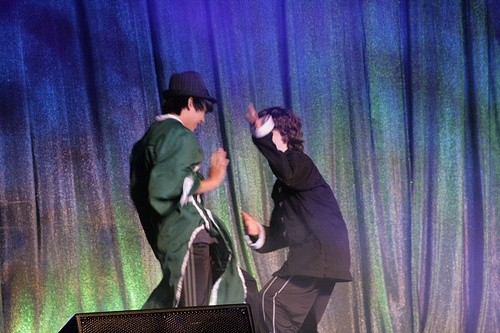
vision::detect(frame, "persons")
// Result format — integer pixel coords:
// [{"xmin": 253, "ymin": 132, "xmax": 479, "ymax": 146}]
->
[
  {"xmin": 128, "ymin": 70, "xmax": 232, "ymax": 309},
  {"xmin": 241, "ymin": 102, "xmax": 350, "ymax": 333}
]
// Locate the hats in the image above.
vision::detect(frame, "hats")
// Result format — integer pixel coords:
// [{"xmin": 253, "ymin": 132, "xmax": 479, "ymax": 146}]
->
[{"xmin": 163, "ymin": 71, "xmax": 217, "ymax": 104}]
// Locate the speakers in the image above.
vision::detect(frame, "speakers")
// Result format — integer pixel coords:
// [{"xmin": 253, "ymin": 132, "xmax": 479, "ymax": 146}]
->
[{"xmin": 58, "ymin": 304, "xmax": 256, "ymax": 333}]
